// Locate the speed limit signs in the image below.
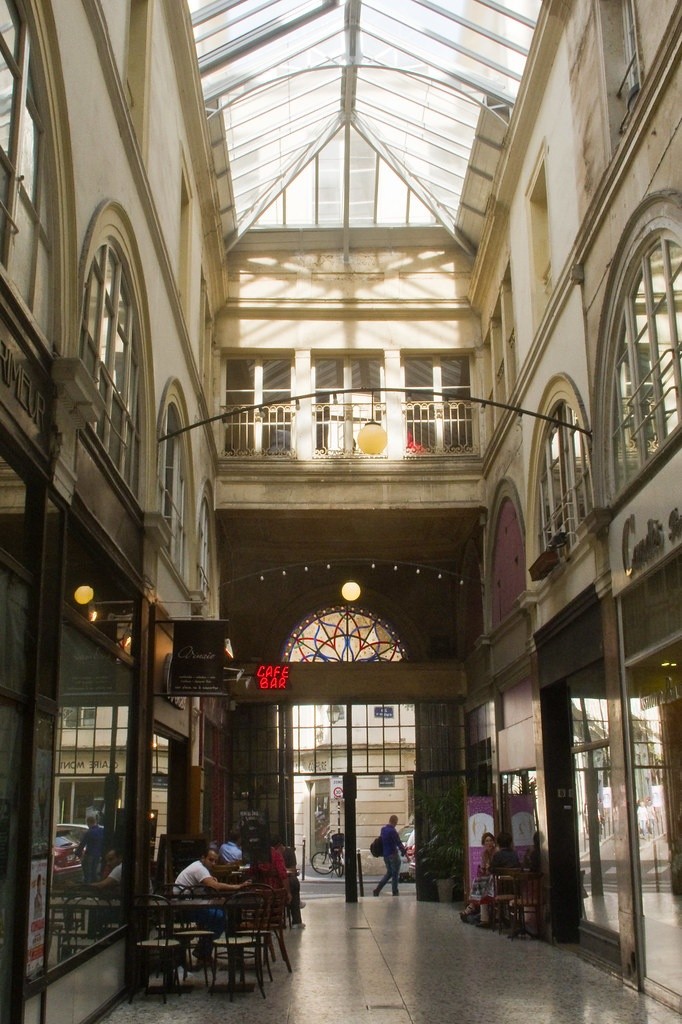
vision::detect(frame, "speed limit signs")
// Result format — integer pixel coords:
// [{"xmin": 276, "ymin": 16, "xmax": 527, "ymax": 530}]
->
[{"xmin": 333, "ymin": 787, "xmax": 343, "ymax": 798}]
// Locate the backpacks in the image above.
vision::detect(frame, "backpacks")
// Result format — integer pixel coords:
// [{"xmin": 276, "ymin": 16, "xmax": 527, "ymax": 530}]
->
[{"xmin": 370, "ymin": 836, "xmax": 383, "ymax": 858}]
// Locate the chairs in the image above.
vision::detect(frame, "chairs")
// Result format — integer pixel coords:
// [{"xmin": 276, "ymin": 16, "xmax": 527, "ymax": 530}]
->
[
  {"xmin": 491, "ymin": 866, "xmax": 542, "ymax": 938},
  {"xmin": 49, "ymin": 884, "xmax": 122, "ymax": 959},
  {"xmin": 135, "ymin": 864, "xmax": 299, "ymax": 1004}
]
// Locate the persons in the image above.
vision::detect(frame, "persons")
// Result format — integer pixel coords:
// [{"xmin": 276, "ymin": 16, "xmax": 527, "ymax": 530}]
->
[
  {"xmin": 168, "ymin": 847, "xmax": 255, "ymax": 964},
  {"xmin": 74, "ymin": 816, "xmax": 104, "ymax": 885},
  {"xmin": 65, "ymin": 846, "xmax": 155, "ymax": 897},
  {"xmin": 219, "ymin": 830, "xmax": 306, "ymax": 929},
  {"xmin": 459, "ymin": 832, "xmax": 521, "ymax": 929},
  {"xmin": 637, "ymin": 800, "xmax": 656, "ymax": 839},
  {"xmin": 372, "ymin": 814, "xmax": 411, "ymax": 897}
]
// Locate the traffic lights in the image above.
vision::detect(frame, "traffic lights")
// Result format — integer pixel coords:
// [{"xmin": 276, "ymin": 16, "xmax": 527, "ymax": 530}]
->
[{"xmin": 149, "ymin": 808, "xmax": 159, "ymax": 842}]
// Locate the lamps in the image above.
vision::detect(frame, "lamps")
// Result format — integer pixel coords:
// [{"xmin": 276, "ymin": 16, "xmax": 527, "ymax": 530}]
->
[
  {"xmin": 356, "ymin": 390, "xmax": 387, "ymax": 455},
  {"xmin": 324, "ymin": 704, "xmax": 341, "ymax": 718},
  {"xmin": 549, "ymin": 532, "xmax": 567, "ymax": 549}
]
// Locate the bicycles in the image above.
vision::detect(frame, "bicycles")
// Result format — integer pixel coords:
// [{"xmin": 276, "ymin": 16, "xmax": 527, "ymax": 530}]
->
[{"xmin": 310, "ymin": 830, "xmax": 344, "ymax": 878}]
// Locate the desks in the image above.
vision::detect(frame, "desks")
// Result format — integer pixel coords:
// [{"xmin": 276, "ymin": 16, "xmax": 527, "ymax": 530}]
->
[
  {"xmin": 49, "ymin": 898, "xmax": 120, "ymax": 911},
  {"xmin": 143, "ymin": 897, "xmax": 260, "ymax": 994},
  {"xmin": 286, "ymin": 869, "xmax": 300, "ymax": 877}
]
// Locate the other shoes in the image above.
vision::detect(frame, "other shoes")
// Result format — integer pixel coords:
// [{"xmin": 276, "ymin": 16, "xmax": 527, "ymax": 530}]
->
[
  {"xmin": 459, "ymin": 912, "xmax": 465, "ymax": 922},
  {"xmin": 291, "ymin": 923, "xmax": 306, "ymax": 929},
  {"xmin": 476, "ymin": 923, "xmax": 489, "ymax": 928},
  {"xmin": 298, "ymin": 902, "xmax": 306, "ymax": 908},
  {"xmin": 374, "ymin": 890, "xmax": 379, "ymax": 896}
]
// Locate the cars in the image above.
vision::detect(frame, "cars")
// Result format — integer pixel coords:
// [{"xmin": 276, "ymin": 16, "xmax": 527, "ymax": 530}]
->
[
  {"xmin": 53, "ymin": 836, "xmax": 87, "ymax": 883},
  {"xmin": 395, "ymin": 825, "xmax": 415, "ymax": 884}
]
